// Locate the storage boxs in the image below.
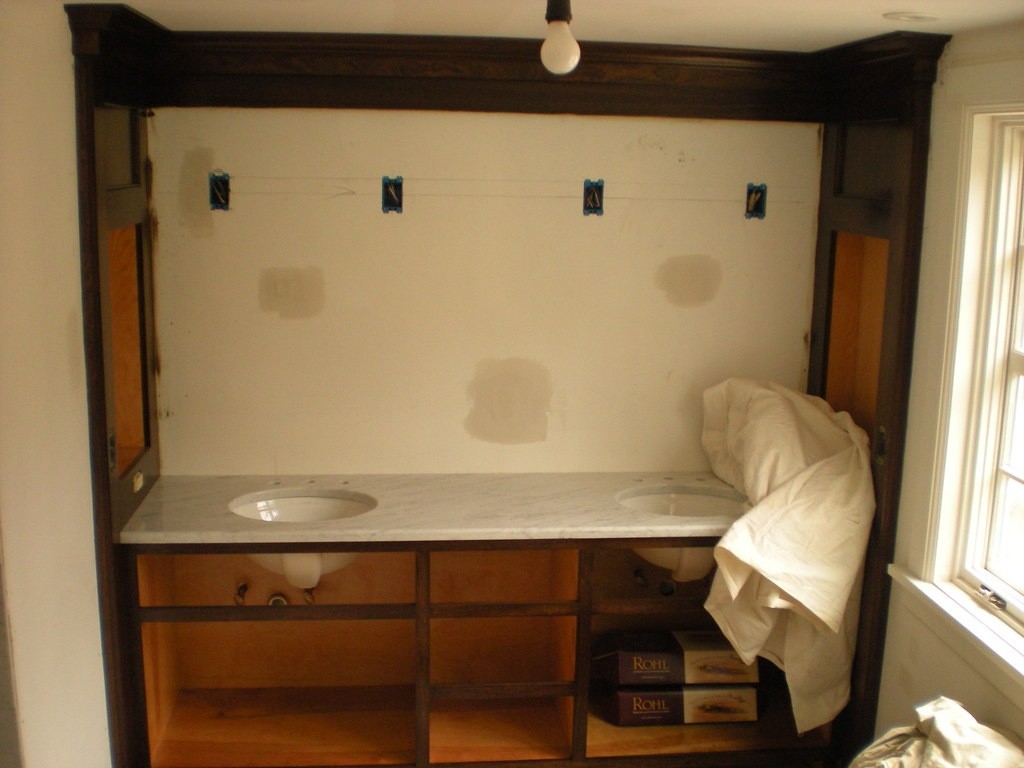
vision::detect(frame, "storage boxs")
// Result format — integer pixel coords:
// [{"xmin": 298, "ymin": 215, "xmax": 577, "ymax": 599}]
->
[
  {"xmin": 589, "ymin": 680, "xmax": 683, "ymax": 726},
  {"xmin": 594, "ymin": 629, "xmax": 685, "ymax": 682},
  {"xmin": 672, "ymin": 628, "xmax": 761, "ymax": 684},
  {"xmin": 683, "ymin": 686, "xmax": 759, "ymax": 723}
]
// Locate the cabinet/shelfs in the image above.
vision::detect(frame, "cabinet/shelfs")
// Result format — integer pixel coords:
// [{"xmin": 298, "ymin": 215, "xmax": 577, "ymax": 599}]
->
[{"xmin": 123, "ymin": 538, "xmax": 891, "ymax": 768}]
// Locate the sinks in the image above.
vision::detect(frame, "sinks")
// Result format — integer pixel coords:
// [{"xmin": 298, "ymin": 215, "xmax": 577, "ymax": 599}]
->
[
  {"xmin": 616, "ymin": 488, "xmax": 751, "ymax": 583},
  {"xmin": 227, "ymin": 485, "xmax": 380, "ymax": 590}
]
